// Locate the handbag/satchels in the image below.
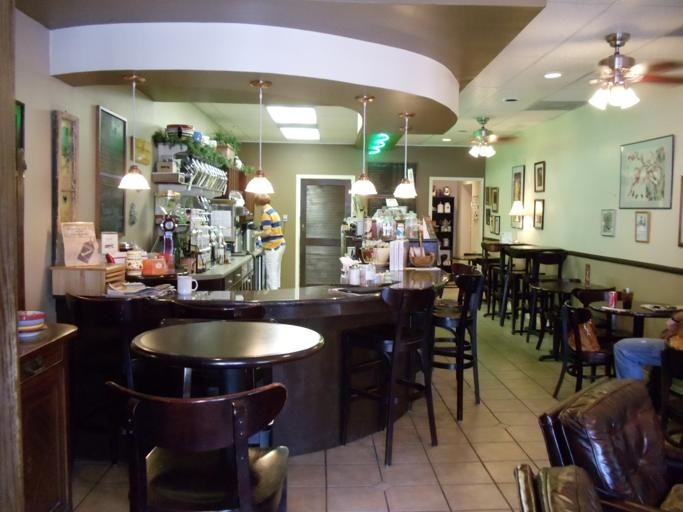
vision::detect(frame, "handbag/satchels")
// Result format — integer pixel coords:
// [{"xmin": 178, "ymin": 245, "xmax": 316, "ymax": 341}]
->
[{"xmin": 567, "ymin": 319, "xmax": 605, "ymax": 353}]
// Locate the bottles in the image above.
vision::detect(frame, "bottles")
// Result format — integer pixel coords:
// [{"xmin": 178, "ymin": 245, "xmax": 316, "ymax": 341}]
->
[
  {"xmin": 77, "ymin": 236, "xmax": 95, "ymax": 264},
  {"xmin": 192, "ymin": 226, "xmax": 229, "ymax": 273}
]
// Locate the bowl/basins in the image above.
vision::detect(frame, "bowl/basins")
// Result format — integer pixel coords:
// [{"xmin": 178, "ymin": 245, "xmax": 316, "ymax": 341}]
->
[{"xmin": 17, "ymin": 311, "xmax": 48, "ymax": 341}]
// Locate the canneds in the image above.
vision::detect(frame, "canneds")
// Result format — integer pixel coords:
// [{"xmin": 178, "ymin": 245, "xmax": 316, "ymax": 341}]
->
[{"xmin": 607, "ymin": 291, "xmax": 618, "ymax": 307}]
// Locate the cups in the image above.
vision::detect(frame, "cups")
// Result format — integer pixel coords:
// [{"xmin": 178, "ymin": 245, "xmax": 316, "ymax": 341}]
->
[
  {"xmin": 347, "ymin": 246, "xmax": 356, "ymax": 258},
  {"xmin": 177, "ymin": 276, "xmax": 198, "ymax": 295},
  {"xmin": 622, "ymin": 288, "xmax": 634, "ymax": 310},
  {"xmin": 340, "ymin": 264, "xmax": 376, "ymax": 287}
]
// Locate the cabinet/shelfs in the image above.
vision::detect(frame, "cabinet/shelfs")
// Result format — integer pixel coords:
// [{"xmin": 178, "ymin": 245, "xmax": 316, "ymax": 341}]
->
[
  {"xmin": 154, "ymin": 142, "xmax": 256, "ymax": 291},
  {"xmin": 432, "ymin": 195, "xmax": 455, "ymax": 273}
]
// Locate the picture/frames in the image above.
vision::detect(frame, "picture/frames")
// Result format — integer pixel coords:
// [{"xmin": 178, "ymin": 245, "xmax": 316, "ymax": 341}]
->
[
  {"xmin": 484, "ymin": 134, "xmax": 683, "ymax": 247},
  {"xmin": 51, "ymin": 110, "xmax": 80, "ymax": 263}
]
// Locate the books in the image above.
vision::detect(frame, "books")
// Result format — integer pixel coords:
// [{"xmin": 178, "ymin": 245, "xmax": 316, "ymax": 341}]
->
[
  {"xmin": 399, "ymin": 240, "xmax": 409, "ymax": 269},
  {"xmin": 389, "ymin": 240, "xmax": 395, "ymax": 271},
  {"xmin": 395, "ymin": 241, "xmax": 399, "ymax": 270}
]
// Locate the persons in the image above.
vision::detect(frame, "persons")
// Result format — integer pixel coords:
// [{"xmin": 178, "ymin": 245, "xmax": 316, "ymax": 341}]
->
[
  {"xmin": 613, "ymin": 310, "xmax": 683, "ymax": 380},
  {"xmin": 253, "ymin": 193, "xmax": 286, "ymax": 290}
]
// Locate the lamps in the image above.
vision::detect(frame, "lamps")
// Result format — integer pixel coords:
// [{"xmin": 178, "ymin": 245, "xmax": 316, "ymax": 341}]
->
[
  {"xmin": 586, "ymin": 32, "xmax": 649, "ymax": 112},
  {"xmin": 468, "ymin": 116, "xmax": 499, "ymax": 160},
  {"xmin": 391, "ymin": 112, "xmax": 418, "ymax": 200},
  {"xmin": 348, "ymin": 94, "xmax": 379, "ymax": 196},
  {"xmin": 242, "ymin": 79, "xmax": 276, "ymax": 196},
  {"xmin": 116, "ymin": 75, "xmax": 152, "ymax": 191}
]
